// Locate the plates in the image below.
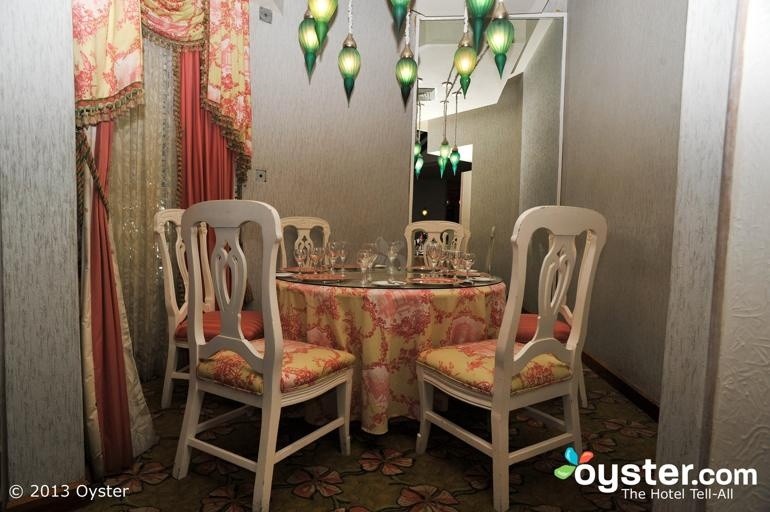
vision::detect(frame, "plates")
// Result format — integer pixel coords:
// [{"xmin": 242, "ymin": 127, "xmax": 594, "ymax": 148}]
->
[
  {"xmin": 444, "ymin": 271, "xmax": 490, "ymax": 278},
  {"xmin": 275, "ymin": 272, "xmax": 293, "ymax": 277},
  {"xmin": 470, "ymin": 276, "xmax": 496, "ymax": 283},
  {"xmin": 287, "ymin": 266, "xmax": 330, "ymax": 273}
]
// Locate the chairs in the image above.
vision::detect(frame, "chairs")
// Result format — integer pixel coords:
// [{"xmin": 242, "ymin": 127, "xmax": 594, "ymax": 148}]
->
[
  {"xmin": 403, "ymin": 220, "xmax": 472, "ymax": 272},
  {"xmin": 277, "ymin": 216, "xmax": 332, "ymax": 269},
  {"xmin": 514, "ymin": 236, "xmax": 592, "ymax": 410},
  {"xmin": 412, "ymin": 205, "xmax": 610, "ymax": 511},
  {"xmin": 170, "ymin": 199, "xmax": 358, "ymax": 510},
  {"xmin": 152, "ymin": 208, "xmax": 264, "ymax": 412}
]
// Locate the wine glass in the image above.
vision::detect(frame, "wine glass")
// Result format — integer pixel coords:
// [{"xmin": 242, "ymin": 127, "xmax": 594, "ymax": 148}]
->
[{"xmin": 291, "ymin": 237, "xmax": 476, "ymax": 286}]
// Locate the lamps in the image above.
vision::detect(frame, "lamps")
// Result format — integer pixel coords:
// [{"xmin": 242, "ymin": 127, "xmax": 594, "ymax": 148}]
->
[
  {"xmin": 297, "ymin": 0, "xmax": 515, "ymax": 112},
  {"xmin": 412, "ymin": 75, "xmax": 462, "ymax": 179}
]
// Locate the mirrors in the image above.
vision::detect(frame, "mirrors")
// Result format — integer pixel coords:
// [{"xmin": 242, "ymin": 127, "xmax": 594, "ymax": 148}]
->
[{"xmin": 405, "ymin": 10, "xmax": 570, "ymax": 315}]
